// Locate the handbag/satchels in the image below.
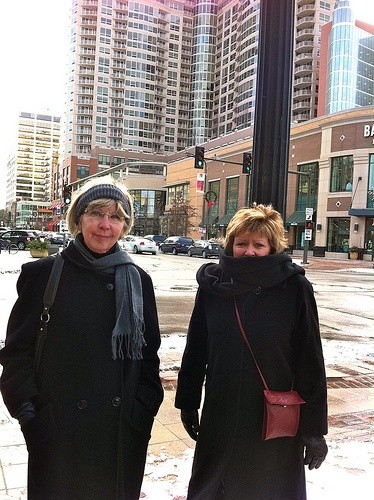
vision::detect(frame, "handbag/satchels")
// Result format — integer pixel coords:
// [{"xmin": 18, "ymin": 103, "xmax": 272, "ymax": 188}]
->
[
  {"xmin": 32, "ymin": 252, "xmax": 65, "ymax": 372},
  {"xmin": 263, "ymin": 389, "xmax": 307, "ymax": 442}
]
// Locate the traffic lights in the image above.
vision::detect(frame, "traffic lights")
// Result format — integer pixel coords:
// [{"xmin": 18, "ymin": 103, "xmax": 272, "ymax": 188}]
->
[
  {"xmin": 306, "ymin": 221, "xmax": 314, "ymax": 228},
  {"xmin": 64, "ymin": 184, "xmax": 72, "ymax": 205},
  {"xmin": 242, "ymin": 152, "xmax": 252, "ymax": 173},
  {"xmin": 194, "ymin": 146, "xmax": 204, "ymax": 169}
]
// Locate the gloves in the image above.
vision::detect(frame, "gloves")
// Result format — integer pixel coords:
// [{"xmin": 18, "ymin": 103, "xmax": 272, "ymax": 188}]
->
[
  {"xmin": 181, "ymin": 408, "xmax": 200, "ymax": 442},
  {"xmin": 297, "ymin": 421, "xmax": 328, "ymax": 471}
]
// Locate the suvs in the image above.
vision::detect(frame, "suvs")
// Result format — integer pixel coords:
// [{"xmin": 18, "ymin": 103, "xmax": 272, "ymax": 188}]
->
[
  {"xmin": 158, "ymin": 235, "xmax": 194, "ymax": 255},
  {"xmin": 0, "ymin": 230, "xmax": 41, "ymax": 250}
]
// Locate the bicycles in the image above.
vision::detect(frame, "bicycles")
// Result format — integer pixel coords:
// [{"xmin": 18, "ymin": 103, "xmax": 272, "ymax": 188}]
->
[{"xmin": 0, "ymin": 238, "xmax": 19, "ymax": 254}]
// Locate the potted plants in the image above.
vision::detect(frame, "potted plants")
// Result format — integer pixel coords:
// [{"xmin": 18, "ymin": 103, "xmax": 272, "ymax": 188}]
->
[
  {"xmin": 25, "ymin": 239, "xmax": 51, "ymax": 258},
  {"xmin": 348, "ymin": 246, "xmax": 359, "ymax": 260}
]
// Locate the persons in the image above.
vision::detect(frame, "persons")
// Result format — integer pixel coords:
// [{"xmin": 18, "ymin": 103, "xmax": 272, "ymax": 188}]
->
[
  {"xmin": 0, "ymin": 174, "xmax": 164, "ymax": 500},
  {"xmin": 175, "ymin": 203, "xmax": 329, "ymax": 500}
]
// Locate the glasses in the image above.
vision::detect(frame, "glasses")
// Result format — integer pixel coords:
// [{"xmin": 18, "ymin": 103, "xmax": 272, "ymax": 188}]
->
[{"xmin": 83, "ymin": 209, "xmax": 122, "ymax": 223}]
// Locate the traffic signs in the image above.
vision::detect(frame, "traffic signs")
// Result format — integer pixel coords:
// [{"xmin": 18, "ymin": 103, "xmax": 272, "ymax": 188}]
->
[
  {"xmin": 306, "ymin": 208, "xmax": 313, "ymax": 220},
  {"xmin": 305, "ymin": 229, "xmax": 312, "ymax": 241}
]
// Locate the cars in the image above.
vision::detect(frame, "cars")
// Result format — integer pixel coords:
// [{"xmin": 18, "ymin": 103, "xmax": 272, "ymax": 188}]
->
[
  {"xmin": 187, "ymin": 240, "xmax": 222, "ymax": 258},
  {"xmin": 119, "ymin": 235, "xmax": 166, "ymax": 255},
  {"xmin": 35, "ymin": 229, "xmax": 71, "ymax": 244}
]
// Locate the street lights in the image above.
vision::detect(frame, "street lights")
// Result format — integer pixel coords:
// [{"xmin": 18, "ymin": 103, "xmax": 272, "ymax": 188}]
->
[{"xmin": 185, "ymin": 151, "xmax": 220, "ymax": 239}]
